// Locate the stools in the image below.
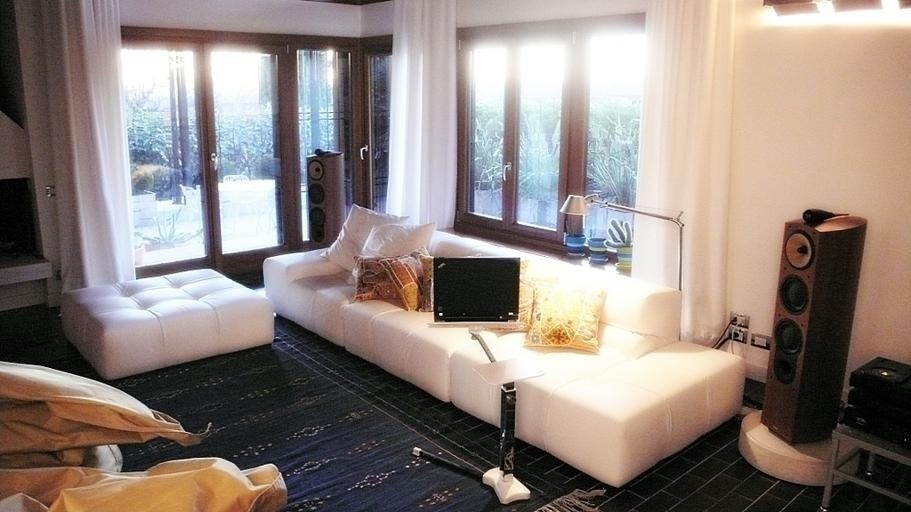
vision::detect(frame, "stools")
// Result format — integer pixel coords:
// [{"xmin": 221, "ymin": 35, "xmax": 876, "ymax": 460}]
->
[{"xmin": 61, "ymin": 267, "xmax": 275, "ymax": 381}]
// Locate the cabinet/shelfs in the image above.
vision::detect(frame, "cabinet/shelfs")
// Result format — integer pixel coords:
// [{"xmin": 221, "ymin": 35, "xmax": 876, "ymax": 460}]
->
[{"xmin": 821, "ymin": 424, "xmax": 911, "ymax": 512}]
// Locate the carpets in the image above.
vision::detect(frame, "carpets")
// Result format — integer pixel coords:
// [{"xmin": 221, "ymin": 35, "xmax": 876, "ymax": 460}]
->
[{"xmin": 29, "ymin": 327, "xmax": 607, "ymax": 512}]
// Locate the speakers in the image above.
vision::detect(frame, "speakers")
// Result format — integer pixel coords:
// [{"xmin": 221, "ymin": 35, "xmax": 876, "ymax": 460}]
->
[
  {"xmin": 758, "ymin": 213, "xmax": 866, "ymax": 446},
  {"xmin": 305, "ymin": 151, "xmax": 347, "ymax": 246}
]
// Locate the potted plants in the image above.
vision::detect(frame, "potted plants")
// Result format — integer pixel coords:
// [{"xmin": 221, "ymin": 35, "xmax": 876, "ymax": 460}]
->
[{"xmin": 604, "ymin": 219, "xmax": 635, "ymax": 272}]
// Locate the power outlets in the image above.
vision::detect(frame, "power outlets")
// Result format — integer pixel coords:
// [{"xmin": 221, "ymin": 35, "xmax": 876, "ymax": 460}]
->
[
  {"xmin": 729, "ymin": 325, "xmax": 747, "ymax": 343},
  {"xmin": 730, "ymin": 312, "xmax": 750, "ymax": 329}
]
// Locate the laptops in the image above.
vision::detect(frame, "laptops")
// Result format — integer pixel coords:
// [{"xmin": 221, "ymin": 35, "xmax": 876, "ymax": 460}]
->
[{"xmin": 432, "ymin": 257, "xmax": 522, "ymax": 323}]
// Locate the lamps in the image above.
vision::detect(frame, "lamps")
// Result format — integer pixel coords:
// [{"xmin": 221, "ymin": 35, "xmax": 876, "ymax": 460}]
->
[{"xmin": 559, "ymin": 193, "xmax": 684, "ymax": 342}]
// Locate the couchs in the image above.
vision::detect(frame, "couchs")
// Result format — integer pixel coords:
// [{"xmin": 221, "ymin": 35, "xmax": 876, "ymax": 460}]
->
[{"xmin": 264, "ymin": 225, "xmax": 746, "ymax": 489}]
